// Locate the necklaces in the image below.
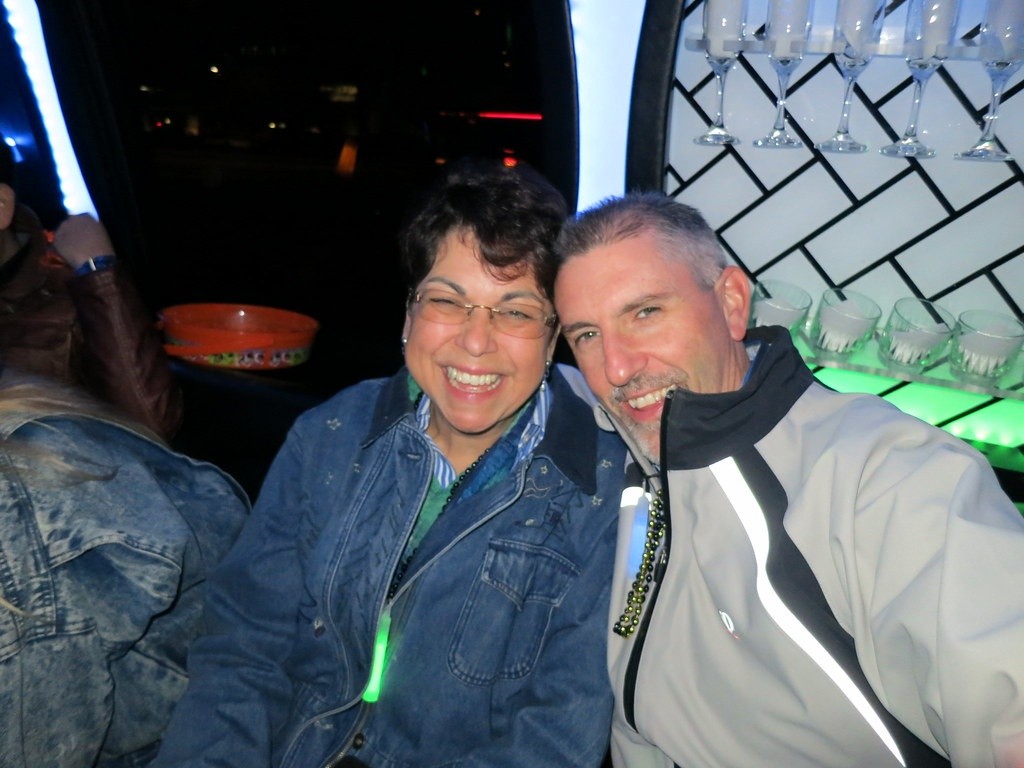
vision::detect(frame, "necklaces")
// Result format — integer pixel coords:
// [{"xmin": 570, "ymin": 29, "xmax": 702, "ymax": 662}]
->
[
  {"xmin": 387, "ymin": 390, "xmax": 490, "ymax": 595},
  {"xmin": 613, "ymin": 490, "xmax": 667, "ymax": 636}
]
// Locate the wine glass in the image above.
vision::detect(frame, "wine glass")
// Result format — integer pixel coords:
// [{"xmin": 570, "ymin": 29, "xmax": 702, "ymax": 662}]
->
[
  {"xmin": 693, "ymin": 0, "xmax": 749, "ymax": 145},
  {"xmin": 814, "ymin": 0, "xmax": 887, "ymax": 153},
  {"xmin": 878, "ymin": 0, "xmax": 963, "ymax": 159},
  {"xmin": 752, "ymin": 0, "xmax": 816, "ymax": 150},
  {"xmin": 954, "ymin": 0, "xmax": 1024, "ymax": 160}
]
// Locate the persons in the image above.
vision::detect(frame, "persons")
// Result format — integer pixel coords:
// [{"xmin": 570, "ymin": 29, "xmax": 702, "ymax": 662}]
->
[
  {"xmin": 553, "ymin": 194, "xmax": 1024, "ymax": 768},
  {"xmin": 0, "ymin": 139, "xmax": 183, "ymax": 437},
  {"xmin": 0, "ymin": 369, "xmax": 252, "ymax": 768},
  {"xmin": 147, "ymin": 166, "xmax": 629, "ymax": 768}
]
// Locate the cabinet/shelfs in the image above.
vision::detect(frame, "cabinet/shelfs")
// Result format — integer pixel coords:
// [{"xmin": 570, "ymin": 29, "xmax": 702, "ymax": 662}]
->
[{"xmin": 682, "ymin": 25, "xmax": 1024, "ymax": 401}]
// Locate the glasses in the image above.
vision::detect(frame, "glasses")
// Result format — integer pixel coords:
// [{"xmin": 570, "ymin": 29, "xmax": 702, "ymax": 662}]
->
[{"xmin": 413, "ymin": 290, "xmax": 557, "ymax": 339}]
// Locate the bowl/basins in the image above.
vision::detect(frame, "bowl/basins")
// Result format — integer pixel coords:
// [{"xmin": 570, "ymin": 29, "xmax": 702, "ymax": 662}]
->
[{"xmin": 157, "ymin": 302, "xmax": 318, "ymax": 370}]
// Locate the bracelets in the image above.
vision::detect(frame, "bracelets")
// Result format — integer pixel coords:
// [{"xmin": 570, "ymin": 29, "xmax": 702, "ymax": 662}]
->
[{"xmin": 77, "ymin": 256, "xmax": 116, "ymax": 277}]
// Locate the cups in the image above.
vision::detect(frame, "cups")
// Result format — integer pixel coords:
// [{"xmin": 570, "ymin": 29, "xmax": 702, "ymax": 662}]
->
[
  {"xmin": 949, "ymin": 309, "xmax": 1024, "ymax": 387},
  {"xmin": 809, "ymin": 289, "xmax": 882, "ymax": 364},
  {"xmin": 877, "ymin": 297, "xmax": 956, "ymax": 375},
  {"xmin": 749, "ymin": 280, "xmax": 813, "ymax": 332}
]
